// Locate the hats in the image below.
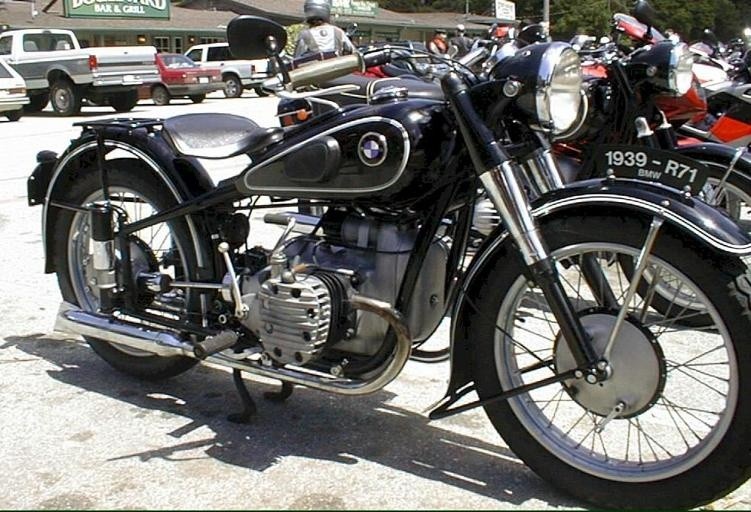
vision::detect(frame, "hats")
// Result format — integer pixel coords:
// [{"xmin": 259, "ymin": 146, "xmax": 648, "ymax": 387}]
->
[{"xmin": 434, "ymin": 28, "xmax": 446, "ymax": 34}]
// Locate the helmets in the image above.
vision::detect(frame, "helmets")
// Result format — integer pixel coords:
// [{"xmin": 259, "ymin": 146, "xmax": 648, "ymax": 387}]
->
[{"xmin": 303, "ymin": 0, "xmax": 332, "ymax": 23}]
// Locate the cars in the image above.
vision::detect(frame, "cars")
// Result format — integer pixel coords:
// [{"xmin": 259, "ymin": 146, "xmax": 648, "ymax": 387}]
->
[{"xmin": 1, "ymin": 23, "xmax": 274, "ymax": 117}]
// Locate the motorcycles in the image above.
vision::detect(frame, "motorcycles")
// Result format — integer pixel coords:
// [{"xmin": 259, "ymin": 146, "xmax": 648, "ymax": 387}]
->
[
  {"xmin": 23, "ymin": 13, "xmax": 751, "ymax": 512},
  {"xmin": 272, "ymin": 14, "xmax": 751, "ymax": 334}
]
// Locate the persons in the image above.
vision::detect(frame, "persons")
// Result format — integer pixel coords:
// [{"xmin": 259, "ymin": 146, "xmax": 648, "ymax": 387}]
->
[
  {"xmin": 428, "ymin": 28, "xmax": 450, "ymax": 57},
  {"xmin": 449, "ymin": 24, "xmax": 474, "ymax": 59},
  {"xmin": 291, "ymin": 0, "xmax": 354, "ymax": 59}
]
